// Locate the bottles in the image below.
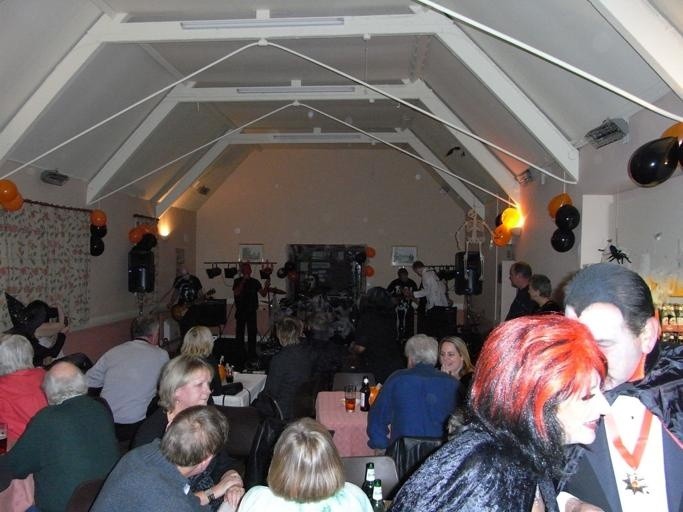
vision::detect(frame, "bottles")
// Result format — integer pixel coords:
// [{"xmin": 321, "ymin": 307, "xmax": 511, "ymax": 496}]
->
[
  {"xmin": 369, "ymin": 479, "xmax": 385, "ymax": 512},
  {"xmin": 359, "ymin": 375, "xmax": 370, "ymax": 411},
  {"xmin": 661, "ymin": 303, "xmax": 683, "ymax": 341},
  {"xmin": 361, "ymin": 462, "xmax": 377, "ymax": 500}
]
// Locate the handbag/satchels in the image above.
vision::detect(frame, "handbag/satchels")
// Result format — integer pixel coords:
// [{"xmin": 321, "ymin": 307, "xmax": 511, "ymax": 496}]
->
[{"xmin": 221, "ymin": 381, "xmax": 243, "ymax": 396}]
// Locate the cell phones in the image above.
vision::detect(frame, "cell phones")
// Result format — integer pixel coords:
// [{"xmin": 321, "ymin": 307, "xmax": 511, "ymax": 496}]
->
[{"xmin": 64, "ymin": 316, "xmax": 68, "ymax": 326}]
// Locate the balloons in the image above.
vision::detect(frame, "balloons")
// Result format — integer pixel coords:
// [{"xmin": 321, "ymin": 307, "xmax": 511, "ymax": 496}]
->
[
  {"xmin": 549, "ymin": 192, "xmax": 579, "ymax": 253},
  {"xmin": 128, "ymin": 223, "xmax": 158, "ymax": 250},
  {"xmin": 90, "ymin": 210, "xmax": 107, "ymax": 257},
  {"xmin": 0, "ymin": 180, "xmax": 24, "ymax": 212},
  {"xmin": 278, "ymin": 260, "xmax": 298, "ymax": 281},
  {"xmin": 355, "ymin": 246, "xmax": 375, "ymax": 276},
  {"xmin": 628, "ymin": 121, "xmax": 683, "ymax": 188},
  {"xmin": 492, "ymin": 207, "xmax": 522, "ymax": 246}
]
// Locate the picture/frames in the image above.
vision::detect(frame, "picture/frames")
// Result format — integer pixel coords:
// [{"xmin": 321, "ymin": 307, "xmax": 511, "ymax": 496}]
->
[
  {"xmin": 239, "ymin": 244, "xmax": 264, "ymax": 262},
  {"xmin": 391, "ymin": 245, "xmax": 418, "ymax": 268}
]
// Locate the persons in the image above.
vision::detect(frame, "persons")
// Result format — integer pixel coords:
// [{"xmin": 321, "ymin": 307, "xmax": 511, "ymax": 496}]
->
[
  {"xmin": 387, "ymin": 313, "xmax": 611, "ymax": 511},
  {"xmin": 547, "ymin": 263, "xmax": 683, "ymax": 512},
  {"xmin": 1, "ymin": 238, "xmax": 567, "ymax": 512}
]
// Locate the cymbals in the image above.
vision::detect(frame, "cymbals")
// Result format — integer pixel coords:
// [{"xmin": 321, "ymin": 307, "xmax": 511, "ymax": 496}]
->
[
  {"xmin": 269, "ymin": 287, "xmax": 287, "ymax": 294},
  {"xmin": 261, "ymin": 300, "xmax": 272, "ymax": 303}
]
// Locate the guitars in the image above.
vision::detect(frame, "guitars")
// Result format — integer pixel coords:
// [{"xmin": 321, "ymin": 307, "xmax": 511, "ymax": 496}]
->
[
  {"xmin": 171, "ymin": 288, "xmax": 216, "ymax": 321},
  {"xmin": 404, "ymin": 289, "xmax": 454, "ymax": 308}
]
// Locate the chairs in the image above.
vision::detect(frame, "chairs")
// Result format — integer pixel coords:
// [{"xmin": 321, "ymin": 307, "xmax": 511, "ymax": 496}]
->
[
  {"xmin": 264, "ymin": 390, "xmax": 285, "ymax": 423},
  {"xmin": 64, "ymin": 478, "xmax": 106, "ymax": 512},
  {"xmin": 332, "ymin": 372, "xmax": 378, "ymax": 391},
  {"xmin": 211, "ymin": 403, "xmax": 268, "ymax": 488},
  {"xmin": 340, "ymin": 456, "xmax": 400, "ymax": 500},
  {"xmin": 384, "ymin": 436, "xmax": 448, "ymax": 478}
]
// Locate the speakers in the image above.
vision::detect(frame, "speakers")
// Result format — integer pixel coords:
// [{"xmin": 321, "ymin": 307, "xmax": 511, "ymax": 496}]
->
[
  {"xmin": 128, "ymin": 252, "xmax": 154, "ymax": 292},
  {"xmin": 181, "ymin": 298, "xmax": 227, "ymax": 327},
  {"xmin": 427, "ymin": 305, "xmax": 457, "ymax": 343},
  {"xmin": 455, "ymin": 251, "xmax": 483, "ymax": 295}
]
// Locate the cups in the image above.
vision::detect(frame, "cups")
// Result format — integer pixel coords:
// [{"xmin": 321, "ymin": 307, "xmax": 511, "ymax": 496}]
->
[
  {"xmin": 225, "ymin": 368, "xmax": 233, "ymax": 383},
  {"xmin": 218, "ymin": 364, "xmax": 225, "ymax": 383},
  {"xmin": 345, "ymin": 384, "xmax": 356, "ymax": 413},
  {"xmin": 0, "ymin": 423, "xmax": 8, "ymax": 455}
]
